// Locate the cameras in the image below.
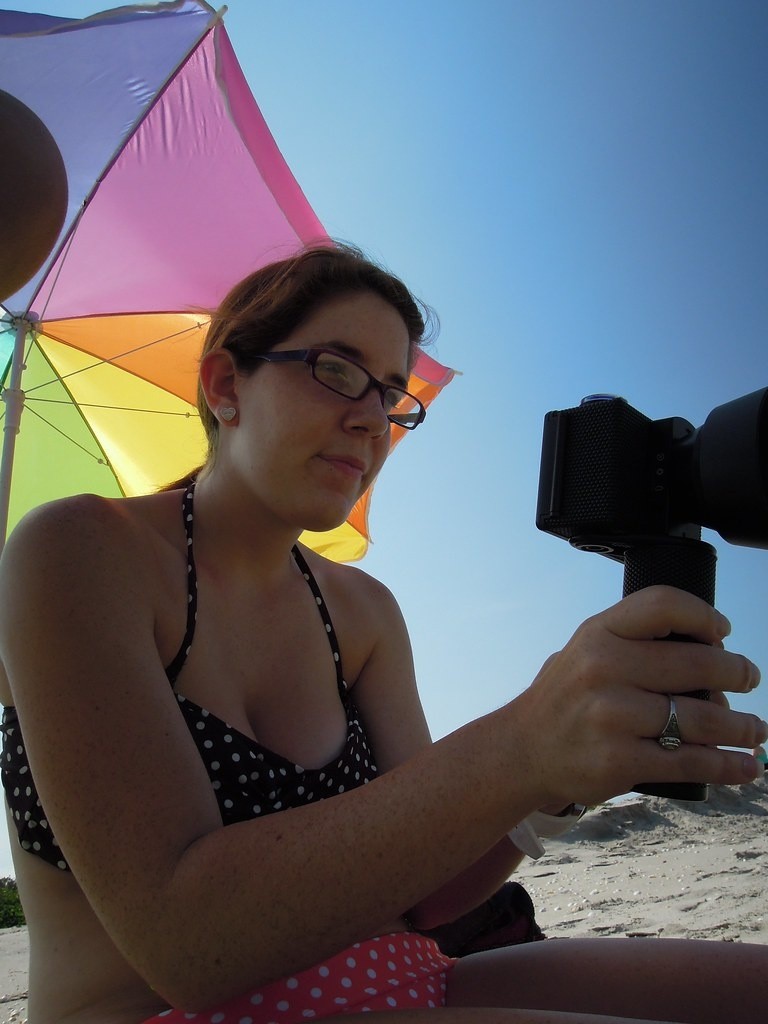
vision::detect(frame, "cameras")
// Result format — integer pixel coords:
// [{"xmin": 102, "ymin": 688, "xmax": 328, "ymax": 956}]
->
[{"xmin": 535, "ymin": 393, "xmax": 768, "ymax": 564}]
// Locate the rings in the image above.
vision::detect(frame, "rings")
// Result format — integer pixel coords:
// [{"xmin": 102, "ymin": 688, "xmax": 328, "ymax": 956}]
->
[{"xmin": 658, "ymin": 696, "xmax": 682, "ymax": 750}]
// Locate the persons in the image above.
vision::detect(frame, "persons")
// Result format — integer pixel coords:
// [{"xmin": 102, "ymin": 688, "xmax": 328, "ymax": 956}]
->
[{"xmin": 0, "ymin": 239, "xmax": 768, "ymax": 1024}]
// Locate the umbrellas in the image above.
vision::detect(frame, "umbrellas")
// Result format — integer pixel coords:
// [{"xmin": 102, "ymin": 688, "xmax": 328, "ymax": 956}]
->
[{"xmin": 0, "ymin": 0, "xmax": 464, "ymax": 566}]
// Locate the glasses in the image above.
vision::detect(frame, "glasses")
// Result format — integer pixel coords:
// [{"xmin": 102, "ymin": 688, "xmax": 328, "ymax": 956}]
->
[{"xmin": 245, "ymin": 348, "xmax": 428, "ymax": 430}]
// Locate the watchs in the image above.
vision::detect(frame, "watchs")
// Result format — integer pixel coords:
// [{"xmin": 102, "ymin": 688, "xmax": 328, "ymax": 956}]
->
[{"xmin": 506, "ymin": 802, "xmax": 587, "ymax": 860}]
